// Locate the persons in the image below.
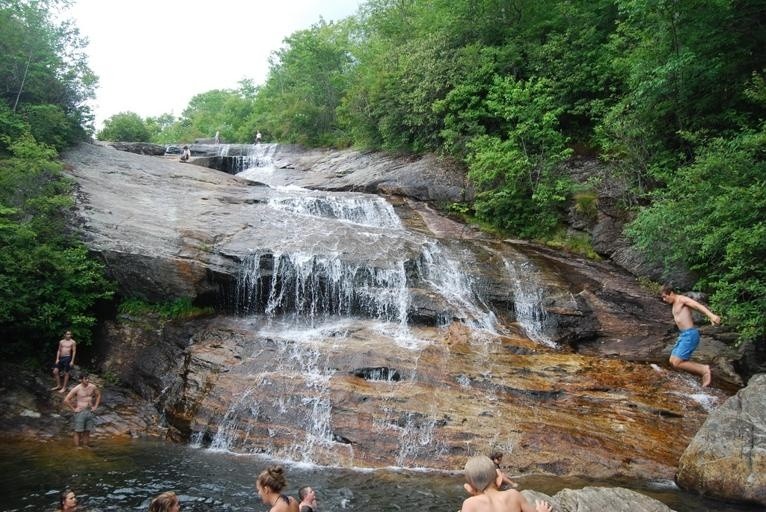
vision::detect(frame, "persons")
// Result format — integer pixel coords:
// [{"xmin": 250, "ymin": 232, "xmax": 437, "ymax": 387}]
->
[
  {"xmin": 254, "ymin": 465, "xmax": 299, "ymax": 512},
  {"xmin": 300, "ymin": 504, "xmax": 312, "ymax": 512},
  {"xmin": 50, "ymin": 329, "xmax": 77, "ymax": 393},
  {"xmin": 660, "ymin": 287, "xmax": 720, "ymax": 387},
  {"xmin": 181, "ymin": 144, "xmax": 191, "ymax": 160},
  {"xmin": 491, "ymin": 451, "xmax": 519, "ymax": 487},
  {"xmin": 212, "ymin": 128, "xmax": 221, "ymax": 144},
  {"xmin": 460, "ymin": 455, "xmax": 554, "ymax": 512},
  {"xmin": 64, "ymin": 372, "xmax": 102, "ymax": 448},
  {"xmin": 255, "ymin": 128, "xmax": 262, "ymax": 143},
  {"xmin": 145, "ymin": 491, "xmax": 180, "ymax": 512},
  {"xmin": 298, "ymin": 486, "xmax": 316, "ymax": 512},
  {"xmin": 54, "ymin": 489, "xmax": 79, "ymax": 512}
]
